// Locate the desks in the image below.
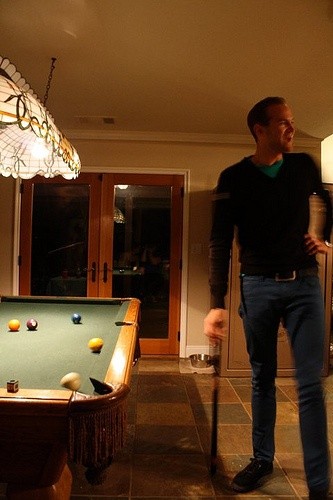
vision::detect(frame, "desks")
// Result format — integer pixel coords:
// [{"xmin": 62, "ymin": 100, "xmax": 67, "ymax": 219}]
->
[
  {"xmin": 112, "ymin": 267, "xmax": 145, "ymax": 278},
  {"xmin": 45, "ymin": 276, "xmax": 88, "ymax": 295},
  {"xmin": 0, "ymin": 294, "xmax": 142, "ymax": 500}
]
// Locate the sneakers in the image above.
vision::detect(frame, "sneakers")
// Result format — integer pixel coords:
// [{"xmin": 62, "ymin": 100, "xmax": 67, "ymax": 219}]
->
[{"xmin": 231, "ymin": 457, "xmax": 273, "ymax": 492}]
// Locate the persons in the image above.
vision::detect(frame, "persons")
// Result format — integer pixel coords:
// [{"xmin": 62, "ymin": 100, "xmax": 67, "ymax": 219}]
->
[{"xmin": 204, "ymin": 96, "xmax": 333, "ymax": 500}]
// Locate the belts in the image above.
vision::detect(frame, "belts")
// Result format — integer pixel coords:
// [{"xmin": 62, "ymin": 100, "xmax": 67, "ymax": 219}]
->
[{"xmin": 240, "ymin": 267, "xmax": 318, "ymax": 281}]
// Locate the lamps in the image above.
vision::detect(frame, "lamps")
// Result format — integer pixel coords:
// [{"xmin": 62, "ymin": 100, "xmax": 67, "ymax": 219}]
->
[
  {"xmin": 0, "ymin": 55, "xmax": 82, "ymax": 180},
  {"xmin": 320, "ymin": 132, "xmax": 333, "ymax": 184}
]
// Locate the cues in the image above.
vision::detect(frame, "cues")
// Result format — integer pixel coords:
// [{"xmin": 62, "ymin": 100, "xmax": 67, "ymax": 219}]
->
[{"xmin": 208, "ymin": 338, "xmax": 221, "ymax": 473}]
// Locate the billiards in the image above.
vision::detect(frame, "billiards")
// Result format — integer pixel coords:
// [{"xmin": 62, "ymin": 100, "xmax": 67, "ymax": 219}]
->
[
  {"xmin": 71, "ymin": 313, "xmax": 82, "ymax": 324},
  {"xmin": 27, "ymin": 319, "xmax": 37, "ymax": 330},
  {"xmin": 60, "ymin": 373, "xmax": 81, "ymax": 392},
  {"xmin": 88, "ymin": 338, "xmax": 103, "ymax": 352},
  {"xmin": 9, "ymin": 320, "xmax": 20, "ymax": 330}
]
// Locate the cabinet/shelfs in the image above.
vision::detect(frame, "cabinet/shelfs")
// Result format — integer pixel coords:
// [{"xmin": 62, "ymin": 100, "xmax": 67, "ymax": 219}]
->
[{"xmin": 210, "ymin": 190, "xmax": 333, "ymax": 377}]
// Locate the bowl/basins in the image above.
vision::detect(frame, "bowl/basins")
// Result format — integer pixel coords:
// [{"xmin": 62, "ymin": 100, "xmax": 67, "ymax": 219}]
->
[{"xmin": 189, "ymin": 354, "xmax": 212, "ymax": 368}]
